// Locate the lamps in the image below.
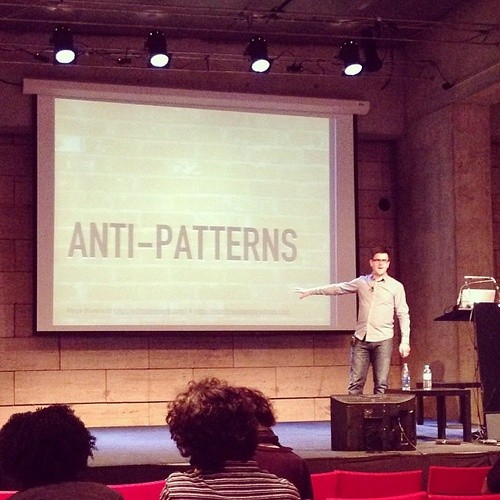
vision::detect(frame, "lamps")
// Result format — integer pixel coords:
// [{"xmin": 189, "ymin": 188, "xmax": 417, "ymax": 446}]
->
[
  {"xmin": 247, "ymin": 35, "xmax": 271, "ymax": 73},
  {"xmin": 338, "ymin": 40, "xmax": 362, "ymax": 76},
  {"xmin": 144, "ymin": 32, "xmax": 170, "ymax": 68},
  {"xmin": 52, "ymin": 26, "xmax": 76, "ymax": 64}
]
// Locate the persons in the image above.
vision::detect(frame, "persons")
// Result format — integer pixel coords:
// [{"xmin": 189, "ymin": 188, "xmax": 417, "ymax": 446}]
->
[
  {"xmin": 159, "ymin": 375, "xmax": 301, "ymax": 500},
  {"xmin": 232, "ymin": 386, "xmax": 314, "ymax": 500},
  {"xmin": 294, "ymin": 248, "xmax": 410, "ymax": 394},
  {"xmin": 0, "ymin": 404, "xmax": 123, "ymax": 500}
]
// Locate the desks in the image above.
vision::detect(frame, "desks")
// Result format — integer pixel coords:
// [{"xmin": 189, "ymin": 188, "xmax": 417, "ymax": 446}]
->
[
  {"xmin": 415, "ymin": 380, "xmax": 481, "ymax": 429},
  {"xmin": 385, "ymin": 388, "xmax": 471, "ymax": 444}
]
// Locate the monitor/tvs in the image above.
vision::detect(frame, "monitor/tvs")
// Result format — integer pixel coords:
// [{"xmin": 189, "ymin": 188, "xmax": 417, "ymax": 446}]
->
[{"xmin": 457, "ymin": 288, "xmax": 496, "ymax": 310}]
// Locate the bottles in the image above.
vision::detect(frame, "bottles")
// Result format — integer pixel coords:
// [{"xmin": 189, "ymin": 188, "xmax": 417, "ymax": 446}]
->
[
  {"xmin": 422, "ymin": 364, "xmax": 431, "ymax": 390},
  {"xmin": 402, "ymin": 363, "xmax": 410, "ymax": 391}
]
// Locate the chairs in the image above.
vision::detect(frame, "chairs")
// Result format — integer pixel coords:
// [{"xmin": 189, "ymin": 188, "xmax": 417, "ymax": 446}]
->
[{"xmin": 0, "ymin": 465, "xmax": 500, "ymax": 500}]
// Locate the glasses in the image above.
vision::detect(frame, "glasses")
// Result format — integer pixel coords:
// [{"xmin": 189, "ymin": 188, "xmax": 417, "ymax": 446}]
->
[{"xmin": 372, "ymin": 259, "xmax": 390, "ymax": 264}]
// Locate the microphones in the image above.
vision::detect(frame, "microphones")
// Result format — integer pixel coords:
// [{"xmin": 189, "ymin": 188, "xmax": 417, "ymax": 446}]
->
[
  {"xmin": 371, "ymin": 286, "xmax": 374, "ymax": 291},
  {"xmin": 464, "ymin": 276, "xmax": 494, "ymax": 280}
]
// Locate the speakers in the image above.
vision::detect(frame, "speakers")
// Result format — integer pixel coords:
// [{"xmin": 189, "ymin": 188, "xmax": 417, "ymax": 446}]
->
[{"xmin": 330, "ymin": 393, "xmax": 417, "ymax": 452}]
